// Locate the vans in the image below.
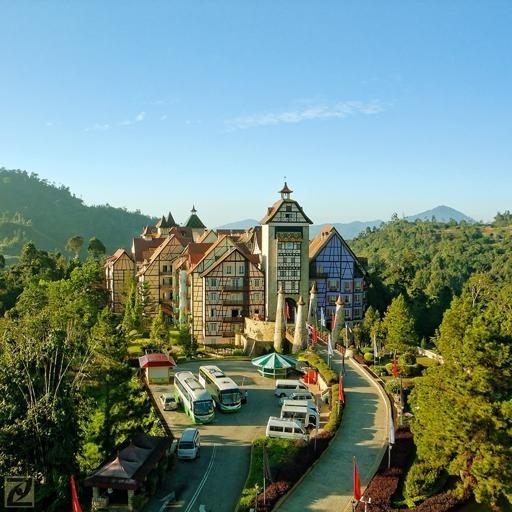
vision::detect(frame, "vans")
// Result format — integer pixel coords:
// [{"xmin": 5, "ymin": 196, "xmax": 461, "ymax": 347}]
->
[{"xmin": 176, "ymin": 427, "xmax": 200, "ymax": 460}]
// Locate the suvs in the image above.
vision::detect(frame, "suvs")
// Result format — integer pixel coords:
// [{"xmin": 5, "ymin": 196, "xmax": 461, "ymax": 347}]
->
[{"xmin": 159, "ymin": 393, "xmax": 178, "ymax": 412}]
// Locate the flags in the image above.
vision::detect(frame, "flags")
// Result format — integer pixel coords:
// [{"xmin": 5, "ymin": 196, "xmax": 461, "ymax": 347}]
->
[
  {"xmin": 393, "ymin": 350, "xmax": 397, "ymax": 378},
  {"xmin": 338, "ymin": 369, "xmax": 344, "ymax": 402},
  {"xmin": 69, "ymin": 475, "xmax": 82, "ymax": 512},
  {"xmin": 328, "ymin": 337, "xmax": 335, "ymax": 357},
  {"xmin": 312, "ymin": 323, "xmax": 316, "ymax": 343},
  {"xmin": 264, "ymin": 448, "xmax": 274, "ymax": 484},
  {"xmin": 353, "ymin": 455, "xmax": 361, "ymax": 501},
  {"xmin": 285, "ymin": 304, "xmax": 291, "ymax": 319}
]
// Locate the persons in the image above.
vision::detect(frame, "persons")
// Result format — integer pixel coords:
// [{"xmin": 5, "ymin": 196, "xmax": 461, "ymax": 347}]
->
[{"xmin": 244, "ymin": 389, "xmax": 248, "ymax": 405}]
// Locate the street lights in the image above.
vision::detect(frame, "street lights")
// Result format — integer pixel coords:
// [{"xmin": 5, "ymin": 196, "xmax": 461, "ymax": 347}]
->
[{"xmin": 359, "ymin": 496, "xmax": 372, "ymax": 512}]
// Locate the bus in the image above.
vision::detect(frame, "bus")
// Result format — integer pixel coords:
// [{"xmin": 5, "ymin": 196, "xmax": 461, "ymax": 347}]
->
[
  {"xmin": 173, "ymin": 370, "xmax": 215, "ymax": 426},
  {"xmin": 198, "ymin": 364, "xmax": 242, "ymax": 414}
]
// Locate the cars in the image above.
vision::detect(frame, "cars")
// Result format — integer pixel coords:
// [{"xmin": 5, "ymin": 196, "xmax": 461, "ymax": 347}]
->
[{"xmin": 265, "ymin": 378, "xmax": 320, "ymax": 440}]
